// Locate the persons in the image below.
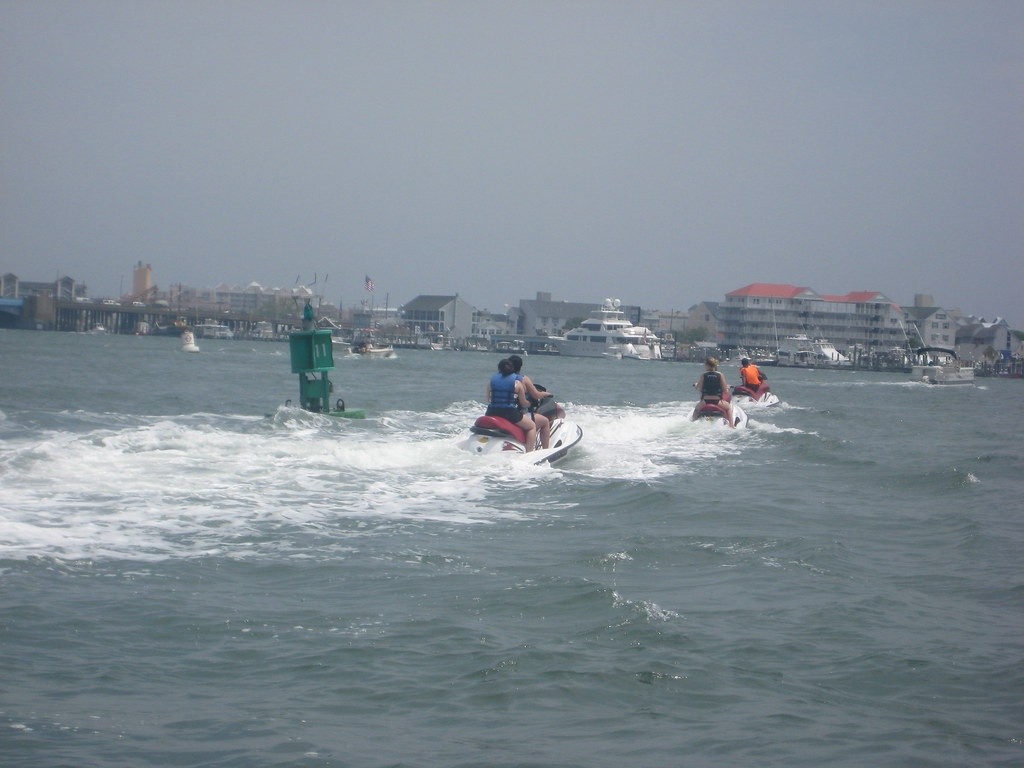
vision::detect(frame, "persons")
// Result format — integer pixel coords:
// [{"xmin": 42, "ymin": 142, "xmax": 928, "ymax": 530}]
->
[
  {"xmin": 741, "ymin": 358, "xmax": 767, "ymax": 392},
  {"xmin": 508, "ymin": 355, "xmax": 551, "ymax": 449},
  {"xmin": 485, "ymin": 359, "xmax": 536, "ymax": 453},
  {"xmin": 692, "ymin": 357, "xmax": 736, "ymax": 428}
]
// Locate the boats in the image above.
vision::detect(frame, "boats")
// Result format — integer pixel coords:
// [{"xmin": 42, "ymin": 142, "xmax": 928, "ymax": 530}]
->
[
  {"xmin": 192, "ymin": 323, "xmax": 234, "ymax": 341},
  {"xmin": 348, "ymin": 327, "xmax": 395, "ymax": 360},
  {"xmin": 419, "ymin": 330, "xmax": 560, "ymax": 357},
  {"xmin": 999, "ymin": 360, "xmax": 1024, "ymax": 380},
  {"xmin": 545, "ymin": 296, "xmax": 669, "ymax": 360},
  {"xmin": 772, "ymin": 318, "xmax": 857, "ymax": 368},
  {"xmin": 897, "ymin": 319, "xmax": 977, "ymax": 387}
]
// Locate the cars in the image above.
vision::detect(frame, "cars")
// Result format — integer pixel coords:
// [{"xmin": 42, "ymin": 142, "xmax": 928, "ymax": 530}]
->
[
  {"xmin": 76, "ymin": 296, "xmax": 93, "ymax": 303},
  {"xmin": 133, "ymin": 301, "xmax": 146, "ymax": 308},
  {"xmin": 103, "ymin": 299, "xmax": 121, "ymax": 306}
]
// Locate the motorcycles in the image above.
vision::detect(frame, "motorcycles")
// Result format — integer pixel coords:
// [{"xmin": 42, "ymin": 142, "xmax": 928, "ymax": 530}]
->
[
  {"xmin": 461, "ymin": 383, "xmax": 583, "ymax": 470},
  {"xmin": 728, "ymin": 378, "xmax": 781, "ymax": 413},
  {"xmin": 689, "ymin": 383, "xmax": 752, "ymax": 434}
]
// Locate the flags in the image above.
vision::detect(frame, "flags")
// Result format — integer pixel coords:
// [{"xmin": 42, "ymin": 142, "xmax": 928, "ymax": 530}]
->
[{"xmin": 364, "ymin": 276, "xmax": 374, "ymax": 292}]
